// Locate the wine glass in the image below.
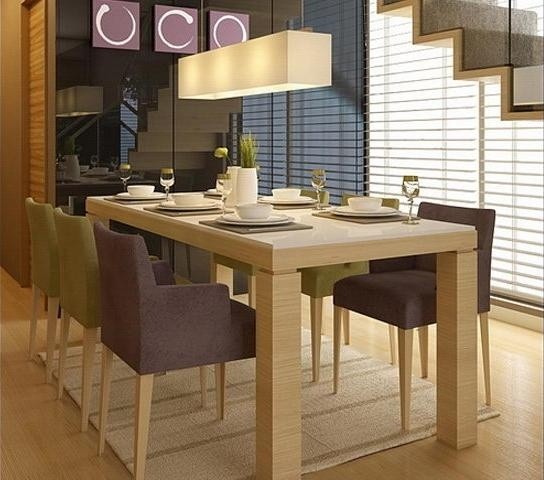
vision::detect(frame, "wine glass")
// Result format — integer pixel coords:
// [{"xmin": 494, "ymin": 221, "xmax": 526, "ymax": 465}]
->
[
  {"xmin": 116, "ymin": 162, "xmax": 132, "ymax": 194},
  {"xmin": 110, "ymin": 156, "xmax": 120, "ymax": 173},
  {"xmin": 310, "ymin": 169, "xmax": 329, "ymax": 211},
  {"xmin": 400, "ymin": 174, "xmax": 422, "ymax": 226},
  {"xmin": 215, "ymin": 174, "xmax": 233, "ymax": 220},
  {"xmin": 159, "ymin": 168, "xmax": 176, "ymax": 206},
  {"xmin": 89, "ymin": 154, "xmax": 100, "ymax": 169}
]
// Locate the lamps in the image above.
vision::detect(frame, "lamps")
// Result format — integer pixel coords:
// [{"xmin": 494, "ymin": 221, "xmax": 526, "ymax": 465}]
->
[
  {"xmin": 177, "ymin": 0, "xmax": 332, "ymax": 100},
  {"xmin": 55, "ymin": 86, "xmax": 103, "ymax": 117}
]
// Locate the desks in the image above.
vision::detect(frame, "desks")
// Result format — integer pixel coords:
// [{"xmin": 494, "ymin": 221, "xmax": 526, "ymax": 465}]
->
[{"xmin": 55, "ymin": 169, "xmax": 161, "ymax": 216}]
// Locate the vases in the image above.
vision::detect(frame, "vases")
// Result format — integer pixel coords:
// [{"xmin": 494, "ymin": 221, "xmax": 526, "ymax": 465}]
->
[{"xmin": 225, "ymin": 166, "xmax": 240, "ymax": 207}]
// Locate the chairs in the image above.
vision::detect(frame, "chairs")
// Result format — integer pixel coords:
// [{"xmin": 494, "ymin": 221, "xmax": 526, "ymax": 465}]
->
[
  {"xmin": 297, "ymin": 197, "xmax": 400, "ymax": 382},
  {"xmin": 332, "ymin": 201, "xmax": 496, "ymax": 433},
  {"xmin": 209, "ymin": 186, "xmax": 330, "ymax": 309},
  {"xmin": 93, "ymin": 221, "xmax": 256, "ymax": 480},
  {"xmin": 25, "ymin": 197, "xmax": 59, "ymax": 384},
  {"xmin": 53, "ymin": 207, "xmax": 206, "ymax": 434}
]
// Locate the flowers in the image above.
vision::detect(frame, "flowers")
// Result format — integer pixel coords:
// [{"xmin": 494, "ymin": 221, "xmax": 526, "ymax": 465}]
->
[{"xmin": 214, "ymin": 145, "xmax": 240, "ymax": 166}]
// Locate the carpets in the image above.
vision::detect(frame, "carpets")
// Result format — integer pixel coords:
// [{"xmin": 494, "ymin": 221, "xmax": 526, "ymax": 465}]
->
[{"xmin": 39, "ymin": 321, "xmax": 501, "ymax": 480}]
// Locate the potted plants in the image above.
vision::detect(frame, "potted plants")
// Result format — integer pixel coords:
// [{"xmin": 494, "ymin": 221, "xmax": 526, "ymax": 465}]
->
[
  {"xmin": 236, "ymin": 129, "xmax": 258, "ymax": 207},
  {"xmin": 64, "ymin": 137, "xmax": 81, "ymax": 179}
]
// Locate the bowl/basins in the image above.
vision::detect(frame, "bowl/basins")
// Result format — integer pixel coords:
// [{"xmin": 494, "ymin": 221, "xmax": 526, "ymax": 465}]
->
[
  {"xmin": 127, "ymin": 184, "xmax": 302, "ymax": 201},
  {"xmin": 94, "ymin": 167, "xmax": 108, "ymax": 175},
  {"xmin": 79, "ymin": 165, "xmax": 89, "ymax": 172}
]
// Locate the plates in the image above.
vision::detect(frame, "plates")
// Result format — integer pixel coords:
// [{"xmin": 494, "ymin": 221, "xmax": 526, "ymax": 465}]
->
[{"xmin": 116, "ymin": 191, "xmax": 400, "ymax": 227}]
